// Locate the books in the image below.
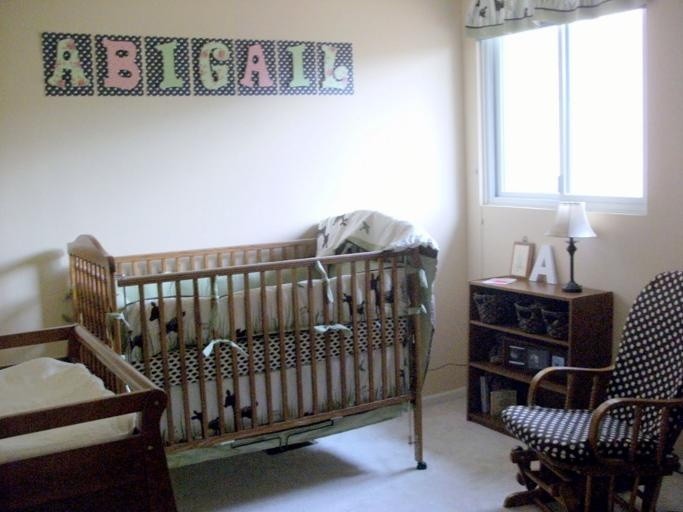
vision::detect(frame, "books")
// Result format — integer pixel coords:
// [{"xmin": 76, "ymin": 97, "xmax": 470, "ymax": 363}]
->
[{"xmin": 479, "ymin": 374, "xmax": 516, "ymax": 417}]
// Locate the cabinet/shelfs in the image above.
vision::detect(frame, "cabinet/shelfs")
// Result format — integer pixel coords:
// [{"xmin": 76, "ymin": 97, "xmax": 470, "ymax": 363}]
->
[{"xmin": 466, "ymin": 274, "xmax": 614, "ymax": 443}]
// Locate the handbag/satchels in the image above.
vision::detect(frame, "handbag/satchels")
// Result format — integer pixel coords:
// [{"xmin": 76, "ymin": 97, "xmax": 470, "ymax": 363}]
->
[
  {"xmin": 514, "ymin": 297, "xmax": 568, "ymax": 340},
  {"xmin": 473, "ymin": 291, "xmax": 518, "ymax": 325}
]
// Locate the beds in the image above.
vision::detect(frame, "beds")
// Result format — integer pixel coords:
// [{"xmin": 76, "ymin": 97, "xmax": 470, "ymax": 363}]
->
[
  {"xmin": 61, "ymin": 209, "xmax": 440, "ymax": 470},
  {"xmin": 0, "ymin": 322, "xmax": 176, "ymax": 512}
]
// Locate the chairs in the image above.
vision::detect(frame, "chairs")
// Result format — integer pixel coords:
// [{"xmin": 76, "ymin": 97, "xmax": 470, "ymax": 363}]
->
[{"xmin": 500, "ymin": 270, "xmax": 683, "ymax": 512}]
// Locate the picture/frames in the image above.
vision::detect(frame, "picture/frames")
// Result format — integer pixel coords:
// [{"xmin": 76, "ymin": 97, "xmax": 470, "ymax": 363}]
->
[{"xmin": 510, "ymin": 241, "xmax": 535, "ymax": 280}]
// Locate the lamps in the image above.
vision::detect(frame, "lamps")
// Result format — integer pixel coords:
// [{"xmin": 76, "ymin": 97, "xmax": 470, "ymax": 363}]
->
[{"xmin": 544, "ymin": 202, "xmax": 598, "ymax": 293}]
